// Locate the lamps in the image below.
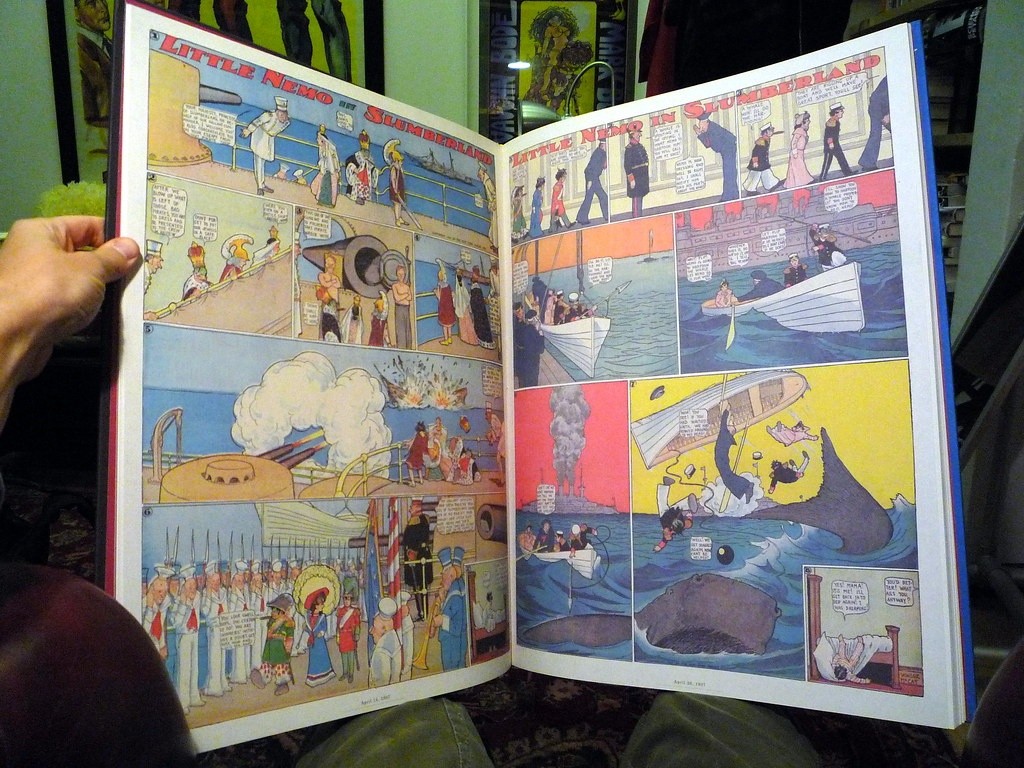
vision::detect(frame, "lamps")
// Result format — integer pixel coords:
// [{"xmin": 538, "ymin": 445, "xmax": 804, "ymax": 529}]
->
[{"xmin": 518, "ymin": 61, "xmax": 615, "ymax": 137}]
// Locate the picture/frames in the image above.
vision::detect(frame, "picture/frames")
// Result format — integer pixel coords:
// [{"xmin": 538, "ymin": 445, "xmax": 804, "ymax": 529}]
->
[
  {"xmin": 46, "ymin": 0, "xmax": 387, "ymax": 189},
  {"xmin": 466, "ymin": 0, "xmax": 638, "ymax": 144}
]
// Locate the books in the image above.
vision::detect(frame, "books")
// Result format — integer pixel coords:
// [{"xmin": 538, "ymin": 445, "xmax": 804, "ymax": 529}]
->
[{"xmin": 99, "ymin": 3, "xmax": 976, "ymax": 732}]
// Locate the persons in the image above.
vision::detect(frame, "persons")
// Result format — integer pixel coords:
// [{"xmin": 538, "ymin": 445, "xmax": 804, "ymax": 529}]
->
[{"xmin": 0, "ymin": 211, "xmax": 823, "ymax": 768}]
